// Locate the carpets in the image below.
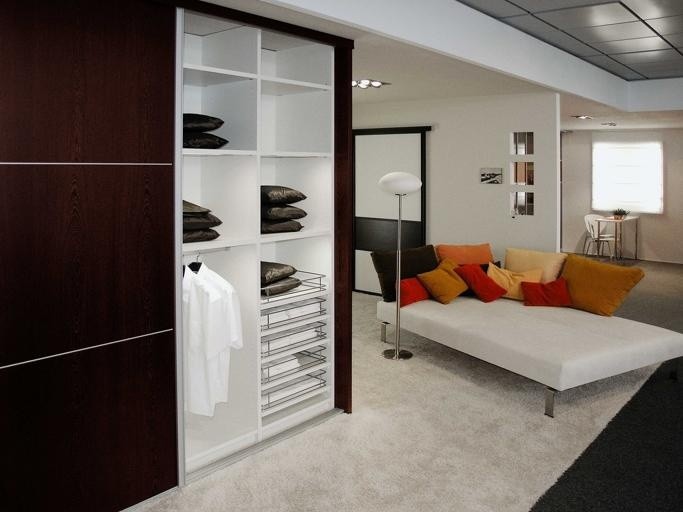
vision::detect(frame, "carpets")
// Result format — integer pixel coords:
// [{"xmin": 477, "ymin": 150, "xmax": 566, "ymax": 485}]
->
[{"xmin": 129, "ymin": 290, "xmax": 665, "ymax": 512}]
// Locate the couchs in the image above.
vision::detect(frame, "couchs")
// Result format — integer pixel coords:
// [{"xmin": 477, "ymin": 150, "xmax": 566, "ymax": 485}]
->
[{"xmin": 371, "ymin": 246, "xmax": 682, "ymax": 417}]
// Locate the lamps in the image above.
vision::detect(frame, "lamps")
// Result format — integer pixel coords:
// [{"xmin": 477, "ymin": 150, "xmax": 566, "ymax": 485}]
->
[{"xmin": 378, "ymin": 171, "xmax": 423, "ymax": 359}]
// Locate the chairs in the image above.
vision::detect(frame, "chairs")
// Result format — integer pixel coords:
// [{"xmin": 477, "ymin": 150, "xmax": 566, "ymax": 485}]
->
[{"xmin": 583, "ymin": 213, "xmax": 620, "ymax": 260}]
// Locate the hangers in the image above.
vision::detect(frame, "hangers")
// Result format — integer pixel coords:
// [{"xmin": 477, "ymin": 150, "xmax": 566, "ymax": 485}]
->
[{"xmin": 181, "ymin": 249, "xmax": 202, "ymax": 277}]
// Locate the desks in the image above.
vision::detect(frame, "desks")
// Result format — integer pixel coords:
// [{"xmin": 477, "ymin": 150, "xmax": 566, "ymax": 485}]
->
[{"xmin": 595, "ymin": 215, "xmax": 639, "ymax": 261}]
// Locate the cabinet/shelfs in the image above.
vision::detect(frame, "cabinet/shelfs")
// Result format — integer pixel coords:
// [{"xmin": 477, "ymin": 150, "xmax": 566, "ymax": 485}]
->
[{"xmin": 174, "ymin": 10, "xmax": 335, "ymax": 485}]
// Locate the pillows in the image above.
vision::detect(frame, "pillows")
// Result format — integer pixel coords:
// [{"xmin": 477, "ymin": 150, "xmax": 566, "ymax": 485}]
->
[
  {"xmin": 371, "ymin": 243, "xmax": 645, "ymax": 315},
  {"xmin": 183, "ymin": 201, "xmax": 222, "ymax": 242},
  {"xmin": 260, "ymin": 183, "xmax": 309, "ymax": 235},
  {"xmin": 183, "ymin": 111, "xmax": 229, "ymax": 149},
  {"xmin": 261, "ymin": 257, "xmax": 304, "ymax": 297}
]
